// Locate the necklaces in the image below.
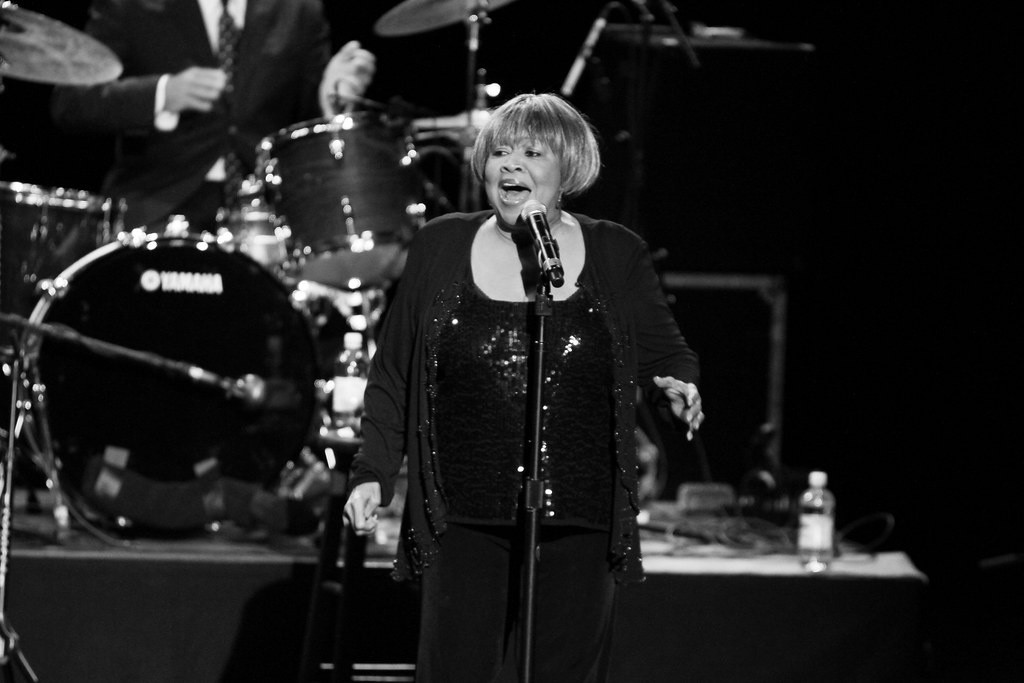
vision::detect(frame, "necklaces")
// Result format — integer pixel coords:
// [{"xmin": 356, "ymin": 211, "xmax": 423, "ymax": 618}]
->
[{"xmin": 496, "ymin": 209, "xmax": 560, "ymax": 240}]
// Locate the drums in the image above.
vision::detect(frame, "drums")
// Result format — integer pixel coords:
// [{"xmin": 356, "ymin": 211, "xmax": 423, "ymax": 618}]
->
[
  {"xmin": 1, "ymin": 182, "xmax": 114, "ymax": 353},
  {"xmin": 17, "ymin": 234, "xmax": 321, "ymax": 544},
  {"xmin": 256, "ymin": 112, "xmax": 427, "ymax": 293}
]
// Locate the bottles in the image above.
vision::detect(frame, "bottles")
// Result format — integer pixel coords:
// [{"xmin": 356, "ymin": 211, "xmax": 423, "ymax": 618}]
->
[
  {"xmin": 798, "ymin": 470, "xmax": 836, "ymax": 574},
  {"xmin": 333, "ymin": 331, "xmax": 371, "ymax": 436}
]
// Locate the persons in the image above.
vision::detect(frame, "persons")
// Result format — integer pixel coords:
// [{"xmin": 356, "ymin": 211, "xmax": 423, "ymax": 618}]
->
[
  {"xmin": 55, "ymin": 1, "xmax": 377, "ymax": 237},
  {"xmin": 342, "ymin": 94, "xmax": 705, "ymax": 683}
]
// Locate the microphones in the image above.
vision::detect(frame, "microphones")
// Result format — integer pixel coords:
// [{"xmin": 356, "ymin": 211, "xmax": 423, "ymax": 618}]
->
[
  {"xmin": 183, "ymin": 362, "xmax": 270, "ymax": 409},
  {"xmin": 521, "ymin": 198, "xmax": 565, "ymax": 288}
]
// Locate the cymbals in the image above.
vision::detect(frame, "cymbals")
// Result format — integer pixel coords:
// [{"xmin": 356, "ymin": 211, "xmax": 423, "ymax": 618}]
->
[
  {"xmin": 0, "ymin": 2, "xmax": 122, "ymax": 88},
  {"xmin": 374, "ymin": 0, "xmax": 517, "ymax": 37}
]
